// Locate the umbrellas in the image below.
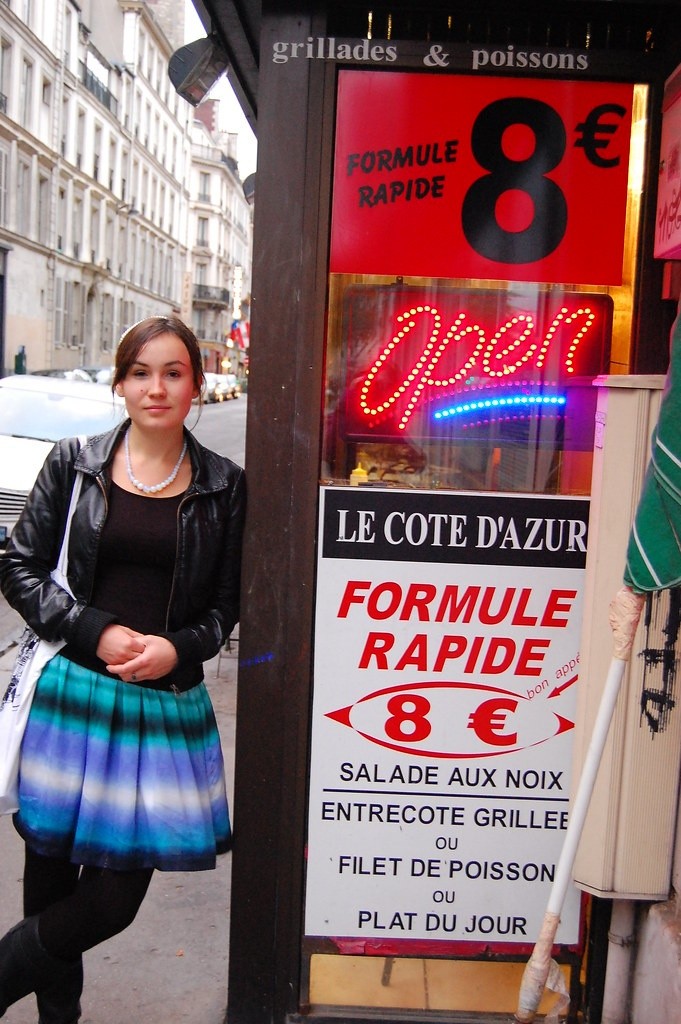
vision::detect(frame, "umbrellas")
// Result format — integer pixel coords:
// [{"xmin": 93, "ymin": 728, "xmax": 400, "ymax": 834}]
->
[{"xmin": 515, "ymin": 309, "xmax": 681, "ymax": 1023}]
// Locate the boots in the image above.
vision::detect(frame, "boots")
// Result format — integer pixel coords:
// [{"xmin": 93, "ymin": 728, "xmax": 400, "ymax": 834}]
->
[
  {"xmin": 0, "ymin": 916, "xmax": 69, "ymax": 1018},
  {"xmin": 38, "ymin": 999, "xmax": 82, "ymax": 1024}
]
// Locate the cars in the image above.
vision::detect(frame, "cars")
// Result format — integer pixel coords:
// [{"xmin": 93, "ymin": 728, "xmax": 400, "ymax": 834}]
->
[
  {"xmin": 191, "ymin": 372, "xmax": 240, "ymax": 405},
  {"xmin": 0, "ymin": 374, "xmax": 129, "ymax": 558},
  {"xmin": 30, "ymin": 366, "xmax": 117, "ymax": 385}
]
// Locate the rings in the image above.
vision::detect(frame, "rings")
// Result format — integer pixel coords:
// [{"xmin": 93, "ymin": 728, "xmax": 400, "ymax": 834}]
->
[{"xmin": 130, "ymin": 672, "xmax": 137, "ymax": 682}]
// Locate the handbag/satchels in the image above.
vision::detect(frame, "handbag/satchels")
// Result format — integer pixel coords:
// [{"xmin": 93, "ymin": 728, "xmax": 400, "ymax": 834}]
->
[{"xmin": 0, "ymin": 571, "xmax": 80, "ymax": 816}]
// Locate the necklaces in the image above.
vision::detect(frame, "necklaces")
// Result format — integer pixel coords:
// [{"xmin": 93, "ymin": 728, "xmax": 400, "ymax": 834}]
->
[{"xmin": 124, "ymin": 426, "xmax": 188, "ymax": 495}]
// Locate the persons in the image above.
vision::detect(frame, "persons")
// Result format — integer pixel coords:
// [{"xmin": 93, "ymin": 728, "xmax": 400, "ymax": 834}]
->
[{"xmin": 0, "ymin": 314, "xmax": 248, "ymax": 1024}]
